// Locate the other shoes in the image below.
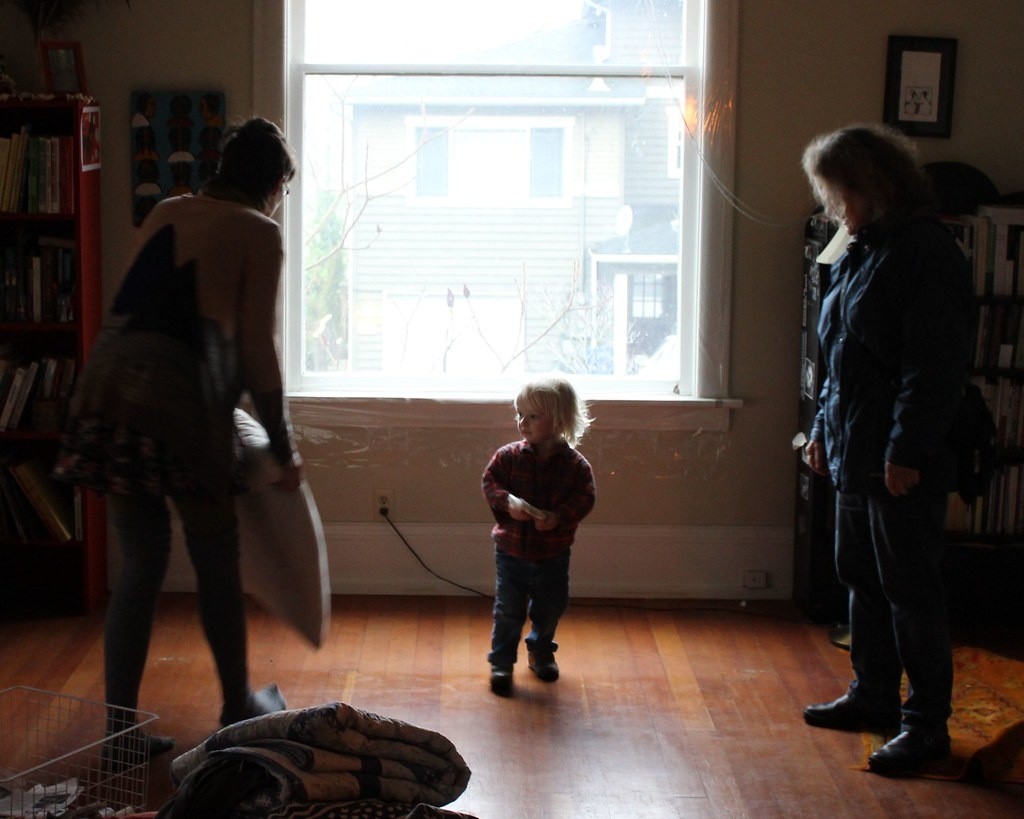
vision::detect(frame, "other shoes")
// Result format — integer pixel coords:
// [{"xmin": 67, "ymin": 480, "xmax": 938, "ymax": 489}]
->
[
  {"xmin": 490, "ymin": 664, "xmax": 513, "ymax": 689},
  {"xmin": 528, "ymin": 651, "xmax": 560, "ymax": 680}
]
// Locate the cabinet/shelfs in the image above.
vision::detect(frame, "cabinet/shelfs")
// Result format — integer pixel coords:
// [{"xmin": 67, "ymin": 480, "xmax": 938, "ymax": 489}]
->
[
  {"xmin": 794, "ymin": 206, "xmax": 1023, "ymax": 625},
  {"xmin": 0, "ymin": 95, "xmax": 108, "ymax": 621}
]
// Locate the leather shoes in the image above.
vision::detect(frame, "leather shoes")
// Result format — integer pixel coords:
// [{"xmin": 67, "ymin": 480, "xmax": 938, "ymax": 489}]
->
[
  {"xmin": 868, "ymin": 728, "xmax": 956, "ymax": 778},
  {"xmin": 800, "ymin": 692, "xmax": 900, "ymax": 731}
]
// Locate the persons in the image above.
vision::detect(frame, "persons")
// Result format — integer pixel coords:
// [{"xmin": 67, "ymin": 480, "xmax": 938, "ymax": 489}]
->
[
  {"xmin": 63, "ymin": 116, "xmax": 307, "ymax": 772},
  {"xmin": 481, "ymin": 385, "xmax": 595, "ymax": 690},
  {"xmin": 798, "ymin": 118, "xmax": 985, "ymax": 774}
]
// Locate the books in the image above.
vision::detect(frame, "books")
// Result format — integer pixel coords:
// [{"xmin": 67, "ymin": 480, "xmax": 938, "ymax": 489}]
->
[
  {"xmin": 938, "ymin": 203, "xmax": 1024, "ymax": 540},
  {"xmin": 0, "ymin": 122, "xmax": 80, "ymax": 540}
]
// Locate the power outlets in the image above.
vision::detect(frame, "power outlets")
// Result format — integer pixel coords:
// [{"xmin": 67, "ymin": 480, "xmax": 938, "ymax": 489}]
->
[{"xmin": 373, "ymin": 489, "xmax": 394, "ymax": 522}]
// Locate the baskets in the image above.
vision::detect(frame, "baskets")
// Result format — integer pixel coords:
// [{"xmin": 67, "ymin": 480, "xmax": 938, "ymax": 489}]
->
[{"xmin": 0, "ymin": 686, "xmax": 160, "ymax": 819}]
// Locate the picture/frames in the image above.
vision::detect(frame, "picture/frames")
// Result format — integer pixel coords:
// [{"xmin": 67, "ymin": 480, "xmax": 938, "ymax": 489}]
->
[
  {"xmin": 38, "ymin": 37, "xmax": 92, "ymax": 96},
  {"xmin": 882, "ymin": 34, "xmax": 953, "ymax": 138}
]
